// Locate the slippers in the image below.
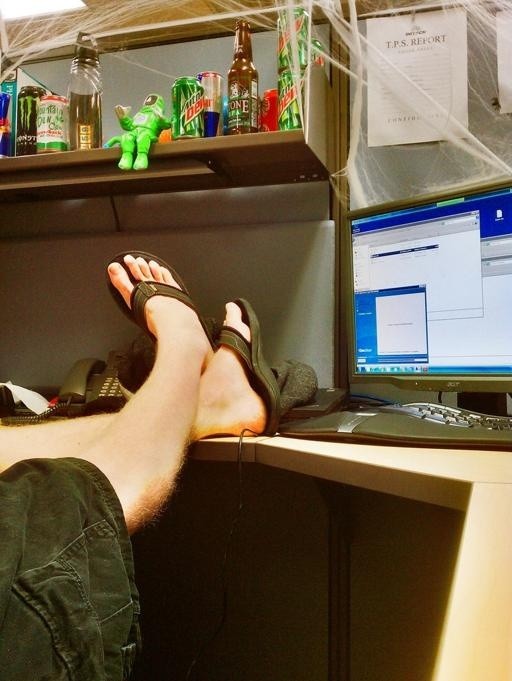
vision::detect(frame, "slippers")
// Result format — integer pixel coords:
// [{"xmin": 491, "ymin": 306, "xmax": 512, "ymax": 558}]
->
[{"xmin": 108, "ymin": 249, "xmax": 283, "ymax": 437}]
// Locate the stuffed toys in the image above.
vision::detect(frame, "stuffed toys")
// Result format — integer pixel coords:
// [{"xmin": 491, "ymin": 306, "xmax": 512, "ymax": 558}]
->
[{"xmin": 104, "ymin": 94, "xmax": 172, "ymax": 171}]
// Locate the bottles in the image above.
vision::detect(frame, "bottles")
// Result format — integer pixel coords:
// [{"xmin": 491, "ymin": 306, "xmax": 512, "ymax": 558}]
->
[
  {"xmin": 68, "ymin": 30, "xmax": 104, "ymax": 149},
  {"xmin": 224, "ymin": 20, "xmax": 261, "ymax": 131}
]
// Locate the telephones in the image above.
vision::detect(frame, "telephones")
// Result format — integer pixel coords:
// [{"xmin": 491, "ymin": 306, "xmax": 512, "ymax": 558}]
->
[{"xmin": 58, "ymin": 350, "xmax": 128, "ymax": 418}]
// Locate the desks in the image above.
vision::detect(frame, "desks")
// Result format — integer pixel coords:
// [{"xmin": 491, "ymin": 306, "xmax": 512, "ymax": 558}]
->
[{"xmin": 183, "ymin": 435, "xmax": 511, "ymax": 681}]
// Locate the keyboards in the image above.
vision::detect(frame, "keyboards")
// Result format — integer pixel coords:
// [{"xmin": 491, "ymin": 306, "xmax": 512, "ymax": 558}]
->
[{"xmin": 281, "ymin": 402, "xmax": 512, "ymax": 448}]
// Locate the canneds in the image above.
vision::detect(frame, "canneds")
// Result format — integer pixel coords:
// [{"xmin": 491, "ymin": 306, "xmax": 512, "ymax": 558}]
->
[
  {"xmin": 256, "ymin": 7, "xmax": 325, "ymax": 133},
  {"xmin": 36, "ymin": 96, "xmax": 70, "ymax": 153},
  {"xmin": 16, "ymin": 85, "xmax": 46, "ymax": 156},
  {"xmin": 0, "ymin": 92, "xmax": 12, "ymax": 159},
  {"xmin": 171, "ymin": 76, "xmax": 204, "ymax": 140}
]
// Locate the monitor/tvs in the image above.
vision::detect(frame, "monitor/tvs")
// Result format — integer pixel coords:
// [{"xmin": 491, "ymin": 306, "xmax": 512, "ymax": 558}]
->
[{"xmin": 343, "ymin": 173, "xmax": 512, "ymax": 416}]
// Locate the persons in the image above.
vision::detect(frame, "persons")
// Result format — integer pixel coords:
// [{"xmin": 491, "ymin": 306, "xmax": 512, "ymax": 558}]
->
[{"xmin": 1, "ymin": 249, "xmax": 280, "ymax": 681}]
